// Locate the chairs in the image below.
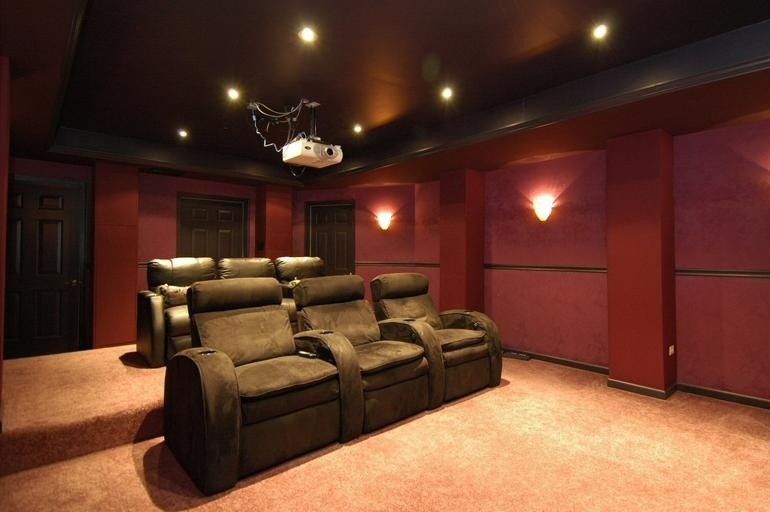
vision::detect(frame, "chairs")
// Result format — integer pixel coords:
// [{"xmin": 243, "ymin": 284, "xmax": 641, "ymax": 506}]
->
[
  {"xmin": 291, "ymin": 273, "xmax": 430, "ymax": 445},
  {"xmin": 368, "ymin": 272, "xmax": 500, "ymax": 411},
  {"xmin": 218, "ymin": 258, "xmax": 278, "ymax": 278},
  {"xmin": 162, "ymin": 275, "xmax": 340, "ymax": 494},
  {"xmin": 274, "ymin": 258, "xmax": 328, "ymax": 278},
  {"xmin": 139, "ymin": 258, "xmax": 220, "ymax": 367}
]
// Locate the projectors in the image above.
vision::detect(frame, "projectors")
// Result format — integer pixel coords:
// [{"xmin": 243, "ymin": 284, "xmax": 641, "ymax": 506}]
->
[{"xmin": 282, "ymin": 139, "xmax": 344, "ymax": 169}]
